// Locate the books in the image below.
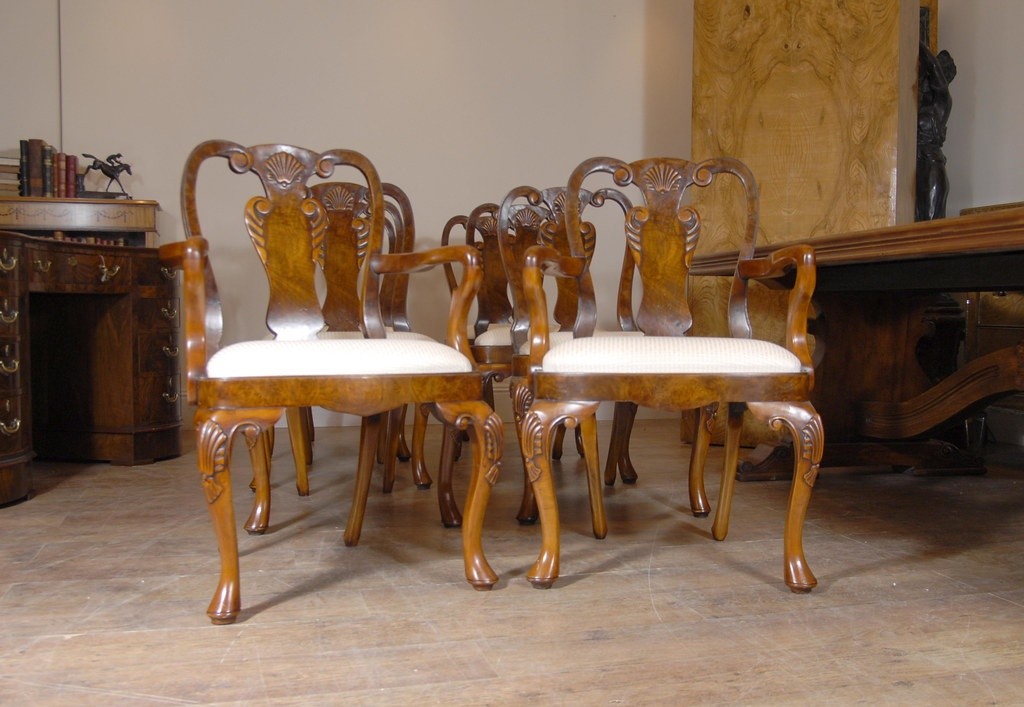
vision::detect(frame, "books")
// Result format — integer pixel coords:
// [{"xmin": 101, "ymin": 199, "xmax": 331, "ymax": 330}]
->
[{"xmin": 0, "ymin": 139, "xmax": 124, "ymax": 246}]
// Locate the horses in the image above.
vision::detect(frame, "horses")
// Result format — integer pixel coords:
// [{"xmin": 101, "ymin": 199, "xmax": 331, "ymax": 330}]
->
[{"xmin": 81, "ymin": 153, "xmax": 132, "ymax": 193}]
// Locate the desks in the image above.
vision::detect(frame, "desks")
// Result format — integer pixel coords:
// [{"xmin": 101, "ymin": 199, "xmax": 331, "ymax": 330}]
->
[{"xmin": 688, "ymin": 207, "xmax": 1024, "ymax": 481}]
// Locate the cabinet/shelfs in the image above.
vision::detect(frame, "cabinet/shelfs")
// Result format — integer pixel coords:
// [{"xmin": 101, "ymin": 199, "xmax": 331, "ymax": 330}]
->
[{"xmin": 0, "ymin": 228, "xmax": 184, "ymax": 510}]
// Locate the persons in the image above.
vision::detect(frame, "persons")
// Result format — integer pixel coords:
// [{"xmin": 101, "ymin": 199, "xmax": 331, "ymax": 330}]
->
[
  {"xmin": 915, "ymin": 40, "xmax": 957, "ymax": 221},
  {"xmin": 107, "ymin": 153, "xmax": 122, "ymax": 167}
]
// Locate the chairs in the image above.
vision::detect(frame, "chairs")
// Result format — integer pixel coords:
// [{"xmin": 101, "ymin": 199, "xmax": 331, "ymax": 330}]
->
[{"xmin": 159, "ymin": 138, "xmax": 826, "ymax": 625}]
